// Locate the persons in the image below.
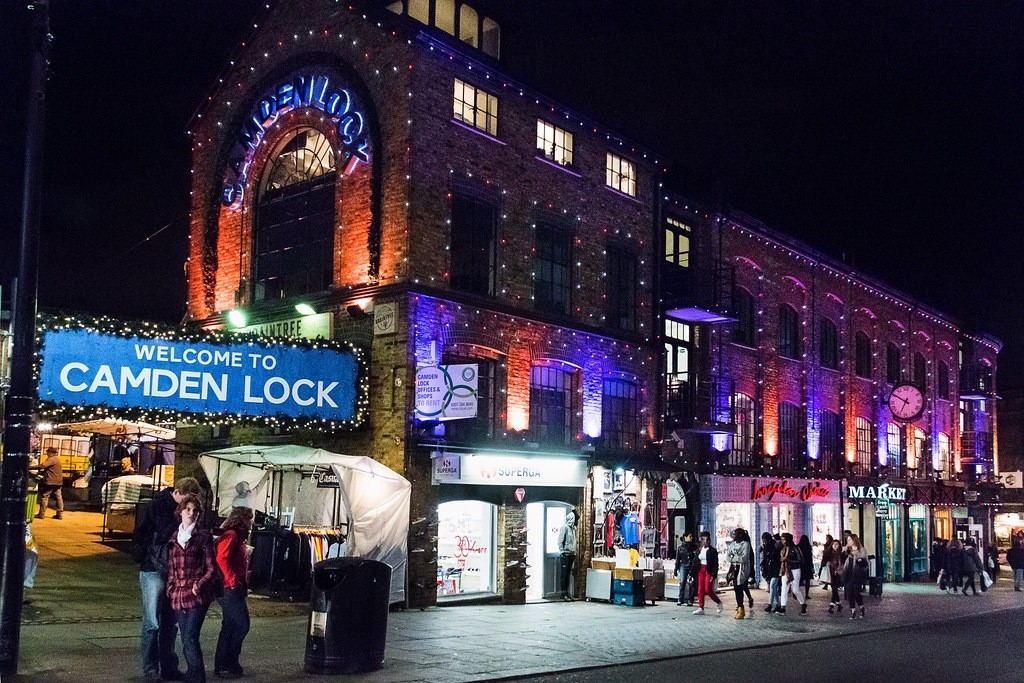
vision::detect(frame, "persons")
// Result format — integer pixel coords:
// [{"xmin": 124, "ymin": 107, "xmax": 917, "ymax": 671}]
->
[
  {"xmin": 556, "ymin": 509, "xmax": 580, "ymax": 602},
  {"xmin": 725, "ymin": 526, "xmax": 756, "ymax": 619},
  {"xmin": 117, "ymin": 456, "xmax": 136, "ymax": 476},
  {"xmin": 929, "ymin": 528, "xmax": 1000, "ymax": 597},
  {"xmin": 209, "ymin": 506, "xmax": 250, "ymax": 679},
  {"xmin": 1005, "ymin": 530, "xmax": 1024, "ymax": 591},
  {"xmin": 792, "ymin": 534, "xmax": 815, "ymax": 600},
  {"xmin": 812, "ymin": 530, "xmax": 868, "ymax": 621},
  {"xmin": 27, "ymin": 446, "xmax": 64, "ymax": 520},
  {"xmin": 164, "ymin": 494, "xmax": 219, "ymax": 683},
  {"xmin": 673, "ymin": 531, "xmax": 723, "ymax": 615},
  {"xmin": 130, "ymin": 477, "xmax": 202, "ymax": 683},
  {"xmin": 231, "ymin": 468, "xmax": 273, "ymax": 518},
  {"xmin": 774, "ymin": 533, "xmax": 807, "ymax": 616},
  {"xmin": 758, "ymin": 531, "xmax": 782, "ymax": 614}
]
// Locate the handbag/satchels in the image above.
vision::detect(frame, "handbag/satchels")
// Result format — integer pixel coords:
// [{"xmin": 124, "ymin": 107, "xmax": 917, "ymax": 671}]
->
[
  {"xmin": 979, "ymin": 570, "xmax": 994, "ymax": 592},
  {"xmin": 819, "ymin": 562, "xmax": 832, "ymax": 584},
  {"xmin": 852, "ymin": 558, "xmax": 870, "ymax": 586},
  {"xmin": 683, "ymin": 577, "xmax": 695, "ymax": 600},
  {"xmin": 936, "ymin": 568, "xmax": 947, "ymax": 590},
  {"xmin": 727, "ymin": 564, "xmax": 735, "ymax": 584}
]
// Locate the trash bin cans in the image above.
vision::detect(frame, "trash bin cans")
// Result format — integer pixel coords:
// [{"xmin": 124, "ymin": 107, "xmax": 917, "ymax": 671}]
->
[{"xmin": 304, "ymin": 556, "xmax": 392, "ymax": 673}]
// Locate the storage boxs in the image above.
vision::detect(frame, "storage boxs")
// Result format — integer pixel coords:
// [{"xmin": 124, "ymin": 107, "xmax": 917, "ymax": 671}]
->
[{"xmin": 592, "ymin": 561, "xmax": 646, "ymax": 607}]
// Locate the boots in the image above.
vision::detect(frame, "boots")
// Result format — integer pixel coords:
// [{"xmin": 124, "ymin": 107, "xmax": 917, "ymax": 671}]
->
[
  {"xmin": 836, "ymin": 600, "xmax": 844, "ymax": 612},
  {"xmin": 735, "ymin": 605, "xmax": 745, "ymax": 619},
  {"xmin": 827, "ymin": 603, "xmax": 836, "ymax": 615}
]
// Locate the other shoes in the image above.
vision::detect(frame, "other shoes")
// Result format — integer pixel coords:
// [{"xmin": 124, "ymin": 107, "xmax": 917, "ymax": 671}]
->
[
  {"xmin": 946, "ymin": 586, "xmax": 954, "ymax": 594},
  {"xmin": 859, "ymin": 606, "xmax": 866, "ymax": 619},
  {"xmin": 779, "ymin": 608, "xmax": 787, "ymax": 616},
  {"xmin": 687, "ymin": 602, "xmax": 693, "ymax": 606},
  {"xmin": 800, "ymin": 603, "xmax": 808, "ymax": 616},
  {"xmin": 160, "ymin": 669, "xmax": 184, "ymax": 680},
  {"xmin": 692, "ymin": 608, "xmax": 705, "ymax": 615},
  {"xmin": 960, "ymin": 591, "xmax": 979, "ymax": 596},
  {"xmin": 749, "ymin": 598, "xmax": 754, "ymax": 608},
  {"xmin": 561, "ymin": 593, "xmax": 575, "ymax": 602},
  {"xmin": 764, "ymin": 604, "xmax": 781, "ymax": 613},
  {"xmin": 144, "ymin": 672, "xmax": 163, "ymax": 683},
  {"xmin": 957, "ymin": 586, "xmax": 964, "ymax": 595},
  {"xmin": 988, "ymin": 556, "xmax": 994, "ymax": 568},
  {"xmin": 677, "ymin": 601, "xmax": 681, "ymax": 606},
  {"xmin": 849, "ymin": 609, "xmax": 856, "ymax": 619},
  {"xmin": 717, "ymin": 602, "xmax": 724, "ymax": 613}
]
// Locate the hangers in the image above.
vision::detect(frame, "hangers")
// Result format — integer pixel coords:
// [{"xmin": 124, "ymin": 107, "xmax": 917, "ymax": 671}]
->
[
  {"xmin": 605, "ymin": 496, "xmax": 639, "ymax": 516},
  {"xmin": 289, "ymin": 524, "xmax": 346, "ymax": 542}
]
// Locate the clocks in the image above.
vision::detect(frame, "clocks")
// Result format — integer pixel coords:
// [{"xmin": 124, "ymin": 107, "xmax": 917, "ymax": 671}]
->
[{"xmin": 889, "ymin": 383, "xmax": 928, "ymax": 421}]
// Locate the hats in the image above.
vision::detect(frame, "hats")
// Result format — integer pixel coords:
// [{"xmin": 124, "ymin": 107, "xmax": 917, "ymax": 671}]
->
[
  {"xmin": 46, "ymin": 447, "xmax": 58, "ymax": 453},
  {"xmin": 780, "ymin": 533, "xmax": 793, "ymax": 539}
]
